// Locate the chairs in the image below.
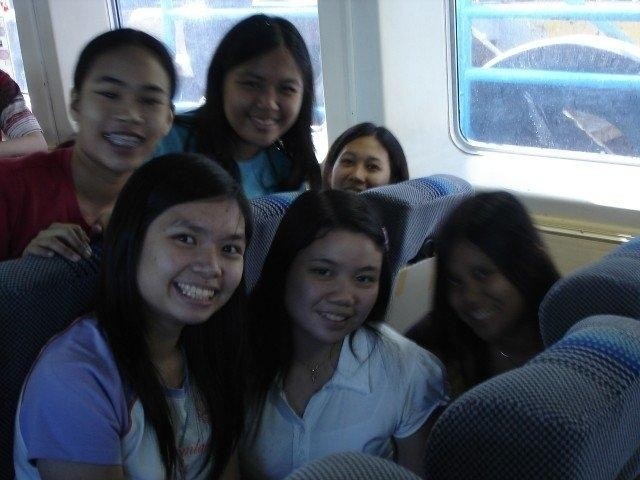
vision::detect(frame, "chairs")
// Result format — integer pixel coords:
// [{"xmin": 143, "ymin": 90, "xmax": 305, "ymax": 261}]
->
[
  {"xmin": 424, "ymin": 316, "xmax": 639, "ymax": 478},
  {"xmin": 284, "ymin": 452, "xmax": 420, "ymax": 478},
  {"xmin": 243, "ymin": 191, "xmax": 303, "ymax": 295},
  {"xmin": 0, "ymin": 245, "xmax": 101, "ymax": 479},
  {"xmin": 355, "ymin": 173, "xmax": 476, "ymax": 335},
  {"xmin": 537, "ymin": 236, "xmax": 640, "ymax": 350}
]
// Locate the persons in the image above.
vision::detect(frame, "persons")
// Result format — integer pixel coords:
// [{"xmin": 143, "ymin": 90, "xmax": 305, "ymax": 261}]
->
[
  {"xmin": 321, "ymin": 121, "xmax": 410, "ymax": 193},
  {"xmin": 0, "ymin": 28, "xmax": 177, "ymax": 263},
  {"xmin": 402, "ymin": 191, "xmax": 562, "ymax": 403},
  {"xmin": 10, "ymin": 152, "xmax": 254, "ymax": 480},
  {"xmin": 0, "ymin": 69, "xmax": 49, "ymax": 157},
  {"xmin": 236, "ymin": 188, "xmax": 452, "ymax": 478},
  {"xmin": 152, "ymin": 13, "xmax": 323, "ymax": 201}
]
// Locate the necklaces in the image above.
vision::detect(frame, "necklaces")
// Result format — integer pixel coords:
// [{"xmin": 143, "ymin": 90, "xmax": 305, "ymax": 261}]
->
[
  {"xmin": 295, "ymin": 345, "xmax": 340, "ymax": 386},
  {"xmin": 492, "ymin": 348, "xmax": 522, "ymax": 368}
]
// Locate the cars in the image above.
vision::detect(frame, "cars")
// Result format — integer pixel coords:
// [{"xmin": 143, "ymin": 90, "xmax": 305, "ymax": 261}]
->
[{"xmin": 481, "ymin": 35, "xmax": 640, "ymax": 75}]
